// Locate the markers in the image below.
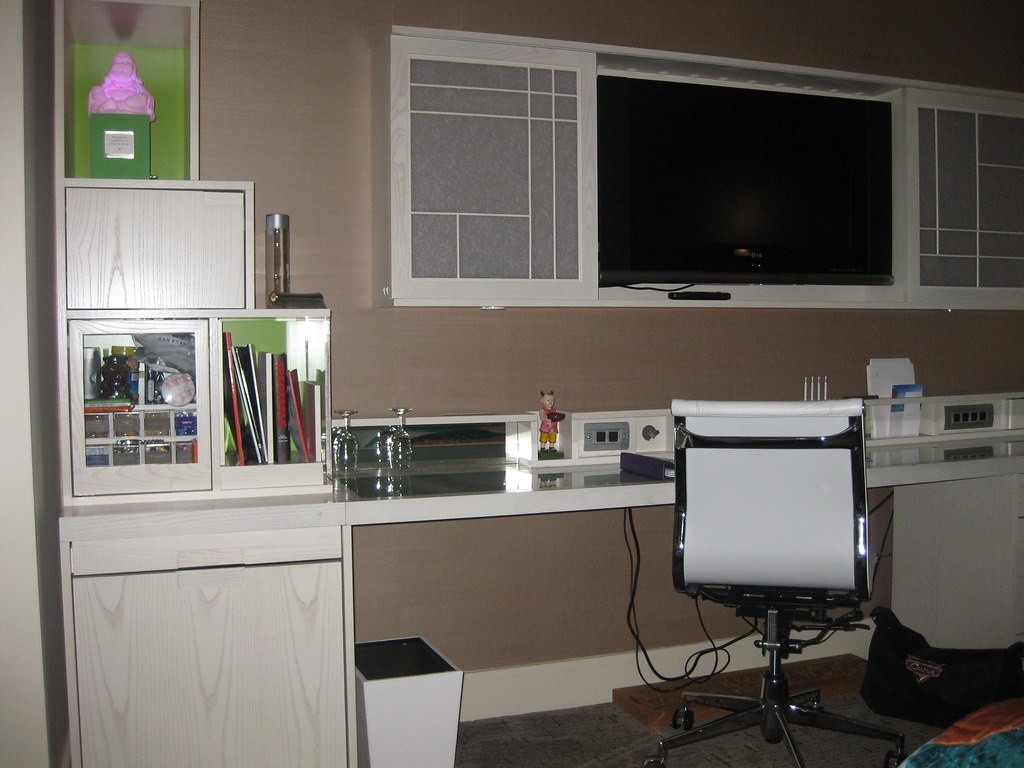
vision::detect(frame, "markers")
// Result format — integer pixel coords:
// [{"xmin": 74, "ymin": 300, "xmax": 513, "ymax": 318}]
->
[{"xmin": 668, "ymin": 292, "xmax": 732, "ymax": 300}]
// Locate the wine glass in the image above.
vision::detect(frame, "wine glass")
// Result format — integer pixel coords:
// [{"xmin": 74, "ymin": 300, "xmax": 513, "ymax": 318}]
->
[
  {"xmin": 386, "ymin": 408, "xmax": 413, "ymax": 468},
  {"xmin": 376, "ymin": 426, "xmax": 396, "ymax": 464},
  {"xmin": 333, "ymin": 409, "xmax": 358, "ymax": 471}
]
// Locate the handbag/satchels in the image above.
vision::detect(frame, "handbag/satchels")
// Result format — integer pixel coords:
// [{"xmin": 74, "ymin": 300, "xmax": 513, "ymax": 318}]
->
[{"xmin": 862, "ymin": 610, "xmax": 1024, "ymax": 730}]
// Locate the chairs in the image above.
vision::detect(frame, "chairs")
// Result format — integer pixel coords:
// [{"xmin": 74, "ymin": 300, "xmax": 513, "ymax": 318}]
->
[{"xmin": 640, "ymin": 394, "xmax": 909, "ymax": 768}]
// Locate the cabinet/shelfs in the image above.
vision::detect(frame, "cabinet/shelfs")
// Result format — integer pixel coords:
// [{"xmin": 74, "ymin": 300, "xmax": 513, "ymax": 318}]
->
[
  {"xmin": 898, "ymin": 78, "xmax": 1024, "ymax": 312},
  {"xmin": 50, "ymin": 2, "xmax": 346, "ymax": 508},
  {"xmin": 388, "ymin": 24, "xmax": 600, "ymax": 306}
]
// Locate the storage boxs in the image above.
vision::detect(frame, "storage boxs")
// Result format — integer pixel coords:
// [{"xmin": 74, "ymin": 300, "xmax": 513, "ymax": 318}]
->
[{"xmin": 612, "ymin": 652, "xmax": 876, "ymax": 727}]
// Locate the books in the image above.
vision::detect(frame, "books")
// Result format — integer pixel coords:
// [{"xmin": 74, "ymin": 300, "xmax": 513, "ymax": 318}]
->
[
  {"xmin": 223, "ymin": 332, "xmax": 321, "ymax": 465},
  {"xmin": 84, "ymin": 398, "xmax": 135, "ymax": 413},
  {"xmin": 620, "ymin": 453, "xmax": 675, "ymax": 479}
]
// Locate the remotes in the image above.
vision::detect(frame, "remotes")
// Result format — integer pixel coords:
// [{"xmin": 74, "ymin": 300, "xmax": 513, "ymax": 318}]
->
[{"xmin": 668, "ymin": 292, "xmax": 732, "ymax": 301}]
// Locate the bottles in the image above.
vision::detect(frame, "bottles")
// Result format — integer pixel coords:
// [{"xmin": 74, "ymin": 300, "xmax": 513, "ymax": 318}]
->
[{"xmin": 100, "ymin": 355, "xmax": 131, "ymax": 401}]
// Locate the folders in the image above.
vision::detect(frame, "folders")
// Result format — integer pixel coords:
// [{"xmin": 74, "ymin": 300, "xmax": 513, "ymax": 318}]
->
[{"xmin": 620, "ymin": 449, "xmax": 676, "ymax": 480}]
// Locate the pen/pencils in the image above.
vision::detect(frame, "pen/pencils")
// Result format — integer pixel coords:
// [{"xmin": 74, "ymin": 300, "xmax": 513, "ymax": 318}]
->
[{"xmin": 803, "ymin": 376, "xmax": 828, "ymax": 400}]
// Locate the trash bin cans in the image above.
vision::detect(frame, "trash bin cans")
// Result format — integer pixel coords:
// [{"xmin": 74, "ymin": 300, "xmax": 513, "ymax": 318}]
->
[{"xmin": 355, "ymin": 636, "xmax": 465, "ymax": 768}]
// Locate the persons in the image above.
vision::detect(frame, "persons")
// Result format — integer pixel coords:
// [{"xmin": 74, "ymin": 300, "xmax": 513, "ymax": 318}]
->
[
  {"xmin": 539, "ymin": 391, "xmax": 560, "ymax": 452},
  {"xmin": 540, "ymin": 479, "xmax": 559, "ymax": 488},
  {"xmin": 89, "ymin": 53, "xmax": 155, "ymax": 122}
]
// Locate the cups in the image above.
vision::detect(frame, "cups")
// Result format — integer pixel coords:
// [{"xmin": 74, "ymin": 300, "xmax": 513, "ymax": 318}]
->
[
  {"xmin": 265, "ymin": 213, "xmax": 291, "ymax": 292},
  {"xmin": 83, "ymin": 347, "xmax": 100, "ymax": 401}
]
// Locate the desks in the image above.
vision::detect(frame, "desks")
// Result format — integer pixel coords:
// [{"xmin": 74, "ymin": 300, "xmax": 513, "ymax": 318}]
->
[{"xmin": 61, "ymin": 453, "xmax": 1023, "ymax": 768}]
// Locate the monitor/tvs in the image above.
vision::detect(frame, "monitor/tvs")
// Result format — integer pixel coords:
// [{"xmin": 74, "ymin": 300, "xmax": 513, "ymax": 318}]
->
[{"xmin": 594, "ymin": 75, "xmax": 894, "ymax": 286}]
